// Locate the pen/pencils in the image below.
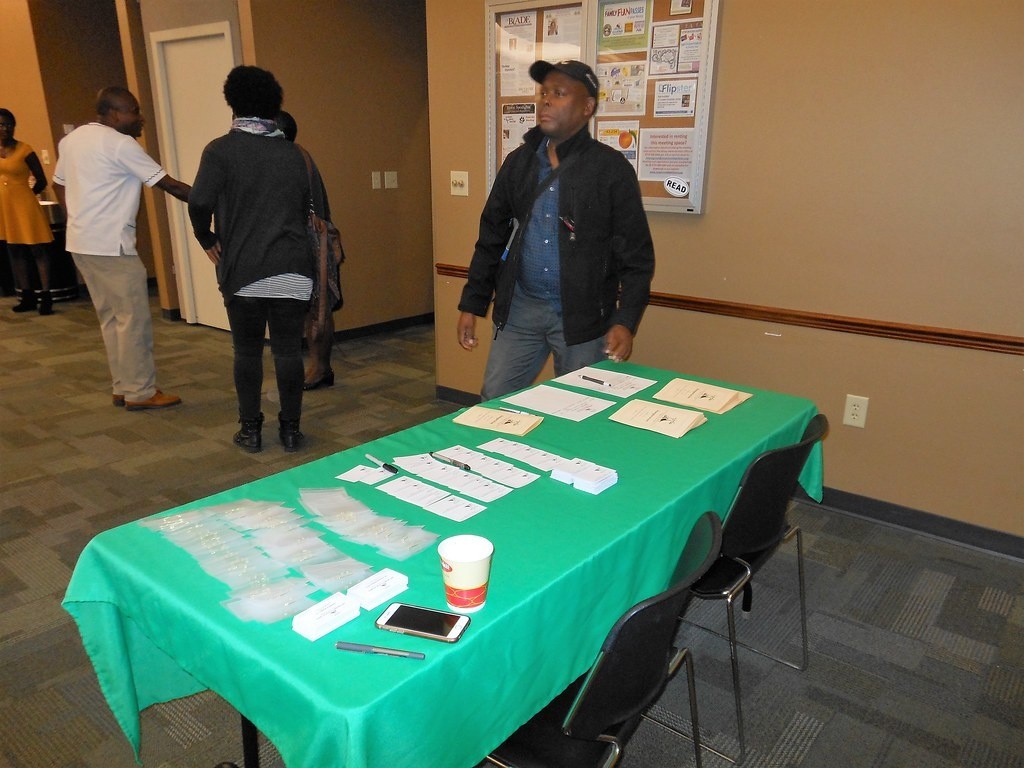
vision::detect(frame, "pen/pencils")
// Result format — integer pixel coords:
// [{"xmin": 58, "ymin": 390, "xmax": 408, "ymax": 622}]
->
[
  {"xmin": 334, "ymin": 642, "xmax": 425, "ymax": 660},
  {"xmin": 430, "ymin": 452, "xmax": 471, "ymax": 470},
  {"xmin": 499, "ymin": 406, "xmax": 534, "ymax": 415},
  {"xmin": 579, "ymin": 374, "xmax": 611, "ymax": 387},
  {"xmin": 365, "ymin": 454, "xmax": 398, "ymax": 474}
]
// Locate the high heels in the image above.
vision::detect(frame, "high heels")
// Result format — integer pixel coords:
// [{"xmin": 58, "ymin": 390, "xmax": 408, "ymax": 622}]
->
[{"xmin": 299, "ymin": 371, "xmax": 334, "ymax": 391}]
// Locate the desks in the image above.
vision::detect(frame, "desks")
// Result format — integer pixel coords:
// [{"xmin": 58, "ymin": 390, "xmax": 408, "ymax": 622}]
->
[{"xmin": 61, "ymin": 360, "xmax": 821, "ymax": 768}]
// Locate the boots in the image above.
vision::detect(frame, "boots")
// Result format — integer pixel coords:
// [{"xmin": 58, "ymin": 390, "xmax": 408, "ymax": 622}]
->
[
  {"xmin": 275, "ymin": 411, "xmax": 301, "ymax": 452},
  {"xmin": 232, "ymin": 414, "xmax": 264, "ymax": 452}
]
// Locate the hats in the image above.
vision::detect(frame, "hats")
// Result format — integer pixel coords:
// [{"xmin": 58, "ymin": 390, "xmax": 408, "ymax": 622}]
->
[{"xmin": 530, "ymin": 59, "xmax": 600, "ymax": 105}]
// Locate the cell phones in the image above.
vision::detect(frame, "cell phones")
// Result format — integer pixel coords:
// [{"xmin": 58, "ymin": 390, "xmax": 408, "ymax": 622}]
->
[{"xmin": 375, "ymin": 602, "xmax": 471, "ymax": 643}]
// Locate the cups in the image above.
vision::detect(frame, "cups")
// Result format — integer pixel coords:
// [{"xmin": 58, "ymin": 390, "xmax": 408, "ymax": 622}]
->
[{"xmin": 437, "ymin": 535, "xmax": 495, "ymax": 615}]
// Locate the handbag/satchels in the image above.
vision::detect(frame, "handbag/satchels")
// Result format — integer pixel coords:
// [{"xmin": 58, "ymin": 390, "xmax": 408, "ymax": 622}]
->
[{"xmin": 297, "ymin": 144, "xmax": 344, "ymax": 343}]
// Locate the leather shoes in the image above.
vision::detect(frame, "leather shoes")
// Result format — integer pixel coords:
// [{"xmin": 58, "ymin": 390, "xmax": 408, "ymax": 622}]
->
[
  {"xmin": 112, "ymin": 387, "xmax": 162, "ymax": 406},
  {"xmin": 126, "ymin": 392, "xmax": 182, "ymax": 412}
]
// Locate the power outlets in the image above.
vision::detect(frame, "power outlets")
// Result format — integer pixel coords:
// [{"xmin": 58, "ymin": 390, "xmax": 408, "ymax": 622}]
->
[{"xmin": 843, "ymin": 393, "xmax": 869, "ymax": 428}]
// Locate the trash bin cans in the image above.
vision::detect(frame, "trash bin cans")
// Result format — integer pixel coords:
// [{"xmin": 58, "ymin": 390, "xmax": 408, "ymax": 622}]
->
[{"xmin": 14, "ymin": 201, "xmax": 83, "ymax": 304}]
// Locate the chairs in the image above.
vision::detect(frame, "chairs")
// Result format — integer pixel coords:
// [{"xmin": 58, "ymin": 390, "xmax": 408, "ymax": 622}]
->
[
  {"xmin": 639, "ymin": 413, "xmax": 828, "ymax": 766},
  {"xmin": 484, "ymin": 510, "xmax": 723, "ymax": 768}
]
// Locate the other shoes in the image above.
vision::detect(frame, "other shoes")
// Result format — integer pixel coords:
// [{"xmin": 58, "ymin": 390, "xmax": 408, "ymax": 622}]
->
[
  {"xmin": 15, "ymin": 291, "xmax": 37, "ymax": 312},
  {"xmin": 36, "ymin": 291, "xmax": 53, "ymax": 314}
]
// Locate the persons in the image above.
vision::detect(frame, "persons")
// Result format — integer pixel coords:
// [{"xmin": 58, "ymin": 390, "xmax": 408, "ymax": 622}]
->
[
  {"xmin": 50, "ymin": 85, "xmax": 193, "ymax": 410},
  {"xmin": 0, "ymin": 106, "xmax": 56, "ymax": 315},
  {"xmin": 262, "ymin": 109, "xmax": 344, "ymax": 388},
  {"xmin": 188, "ymin": 66, "xmax": 324, "ymax": 456},
  {"xmin": 455, "ymin": 61, "xmax": 654, "ymax": 407}
]
